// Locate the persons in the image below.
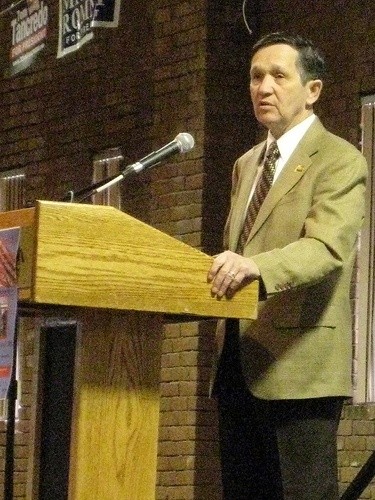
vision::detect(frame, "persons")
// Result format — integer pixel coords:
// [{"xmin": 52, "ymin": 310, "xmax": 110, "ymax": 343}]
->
[{"xmin": 207, "ymin": 32, "xmax": 369, "ymax": 500}]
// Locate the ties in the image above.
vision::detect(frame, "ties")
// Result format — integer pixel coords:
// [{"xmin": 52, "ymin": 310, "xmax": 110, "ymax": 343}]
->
[{"xmin": 233, "ymin": 142, "xmax": 280, "ymax": 255}]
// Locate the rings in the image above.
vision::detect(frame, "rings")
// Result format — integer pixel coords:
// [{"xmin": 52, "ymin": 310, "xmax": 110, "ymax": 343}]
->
[{"xmin": 228, "ymin": 272, "xmax": 235, "ymax": 279}]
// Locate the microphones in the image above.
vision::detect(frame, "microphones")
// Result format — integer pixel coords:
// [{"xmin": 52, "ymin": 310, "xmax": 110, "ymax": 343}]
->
[{"xmin": 122, "ymin": 132, "xmax": 195, "ymax": 177}]
